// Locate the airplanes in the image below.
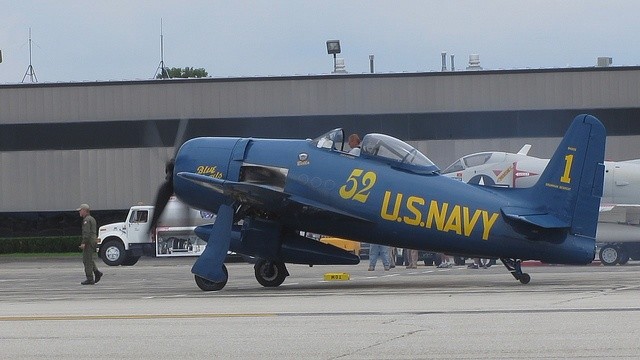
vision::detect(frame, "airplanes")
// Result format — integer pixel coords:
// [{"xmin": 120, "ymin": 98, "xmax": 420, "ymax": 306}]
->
[
  {"xmin": 141, "ymin": 112, "xmax": 605, "ymax": 293},
  {"xmin": 440, "ymin": 140, "xmax": 639, "ymax": 267}
]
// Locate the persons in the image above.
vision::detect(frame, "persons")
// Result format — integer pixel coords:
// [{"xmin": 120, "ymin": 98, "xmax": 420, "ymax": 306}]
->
[
  {"xmin": 347, "ymin": 134, "xmax": 361, "ymax": 157},
  {"xmin": 77, "ymin": 204, "xmax": 103, "ymax": 285},
  {"xmin": 367, "ymin": 243, "xmax": 498, "ymax": 271}
]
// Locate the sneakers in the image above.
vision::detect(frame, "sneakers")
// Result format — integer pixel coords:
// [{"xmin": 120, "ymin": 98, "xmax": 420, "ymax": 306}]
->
[
  {"xmin": 467, "ymin": 263, "xmax": 478, "ymax": 269},
  {"xmin": 95, "ymin": 271, "xmax": 103, "ymax": 283},
  {"xmin": 406, "ymin": 264, "xmax": 417, "ymax": 268},
  {"xmin": 81, "ymin": 279, "xmax": 95, "ymax": 285},
  {"xmin": 436, "ymin": 263, "xmax": 445, "ymax": 268},
  {"xmin": 442, "ymin": 263, "xmax": 451, "ymax": 267},
  {"xmin": 368, "ymin": 267, "xmax": 375, "ymax": 271}
]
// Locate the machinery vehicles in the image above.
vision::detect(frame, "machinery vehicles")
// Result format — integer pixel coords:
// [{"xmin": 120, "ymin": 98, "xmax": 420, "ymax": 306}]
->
[
  {"xmin": 96, "ymin": 196, "xmax": 314, "ymax": 267},
  {"xmin": 391, "ymin": 248, "xmax": 445, "ymax": 266}
]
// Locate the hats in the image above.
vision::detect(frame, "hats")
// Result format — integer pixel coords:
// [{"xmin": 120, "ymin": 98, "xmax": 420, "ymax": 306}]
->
[{"xmin": 76, "ymin": 204, "xmax": 89, "ymax": 211}]
